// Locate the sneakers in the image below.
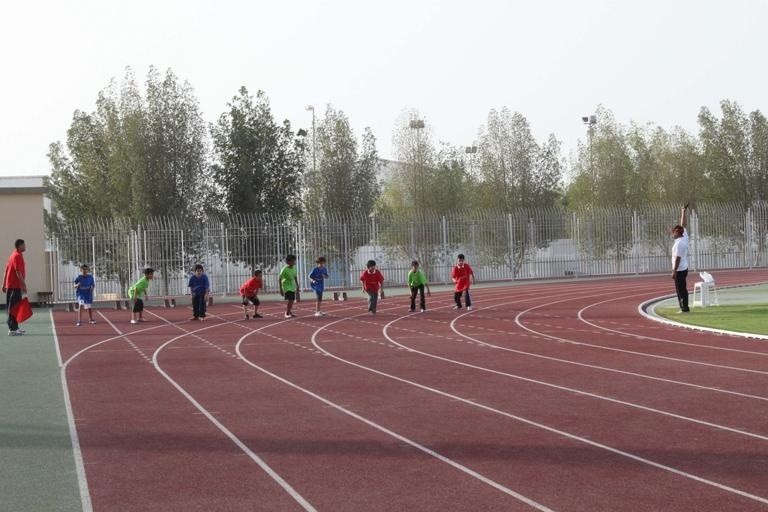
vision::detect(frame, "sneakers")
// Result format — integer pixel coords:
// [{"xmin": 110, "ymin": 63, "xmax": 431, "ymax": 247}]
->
[
  {"xmin": 285, "ymin": 311, "xmax": 296, "ymax": 318},
  {"xmin": 408, "ymin": 309, "xmax": 415, "ymax": 312},
  {"xmin": 131, "ymin": 319, "xmax": 138, "ymax": 323},
  {"xmin": 253, "ymin": 314, "xmax": 263, "ymax": 318},
  {"xmin": 8, "ymin": 330, "xmax": 25, "ymax": 336},
  {"xmin": 467, "ymin": 306, "xmax": 472, "ymax": 311},
  {"xmin": 421, "ymin": 309, "xmax": 425, "ymax": 313},
  {"xmin": 315, "ymin": 311, "xmax": 325, "ymax": 317},
  {"xmin": 89, "ymin": 320, "xmax": 96, "ymax": 324},
  {"xmin": 137, "ymin": 318, "xmax": 145, "ymax": 321},
  {"xmin": 77, "ymin": 322, "xmax": 80, "ymax": 326}
]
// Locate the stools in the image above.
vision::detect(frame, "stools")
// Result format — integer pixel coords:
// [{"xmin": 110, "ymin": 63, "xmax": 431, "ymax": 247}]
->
[
  {"xmin": 693, "ymin": 282, "xmax": 720, "ymax": 309},
  {"xmin": 37, "ymin": 292, "xmax": 53, "ymax": 308}
]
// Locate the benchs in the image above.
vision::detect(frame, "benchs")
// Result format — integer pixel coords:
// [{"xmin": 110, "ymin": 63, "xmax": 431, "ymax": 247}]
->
[{"xmin": 46, "ymin": 283, "xmax": 410, "ymax": 312}]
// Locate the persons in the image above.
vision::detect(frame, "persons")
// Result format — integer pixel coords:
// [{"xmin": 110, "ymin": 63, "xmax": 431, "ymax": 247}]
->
[
  {"xmin": 451, "ymin": 253, "xmax": 477, "ymax": 311},
  {"xmin": 358, "ymin": 260, "xmax": 385, "ymax": 317},
  {"xmin": 407, "ymin": 260, "xmax": 431, "ymax": 312},
  {"xmin": 277, "ymin": 254, "xmax": 300, "ymax": 318},
  {"xmin": 188, "ymin": 264, "xmax": 211, "ymax": 321},
  {"xmin": 2, "ymin": 238, "xmax": 27, "ymax": 336},
  {"xmin": 669, "ymin": 202, "xmax": 692, "ymax": 314},
  {"xmin": 127, "ymin": 268, "xmax": 155, "ymax": 324},
  {"xmin": 238, "ymin": 269, "xmax": 264, "ymax": 320},
  {"xmin": 307, "ymin": 256, "xmax": 329, "ymax": 317},
  {"xmin": 72, "ymin": 264, "xmax": 97, "ymax": 326}
]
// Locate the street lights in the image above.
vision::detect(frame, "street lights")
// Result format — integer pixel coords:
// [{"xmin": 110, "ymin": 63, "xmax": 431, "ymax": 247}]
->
[
  {"xmin": 409, "ymin": 118, "xmax": 424, "ymax": 159},
  {"xmin": 306, "ymin": 103, "xmax": 319, "ymax": 208},
  {"xmin": 464, "ymin": 145, "xmax": 477, "ymax": 179},
  {"xmin": 582, "ymin": 116, "xmax": 596, "ymax": 212}
]
